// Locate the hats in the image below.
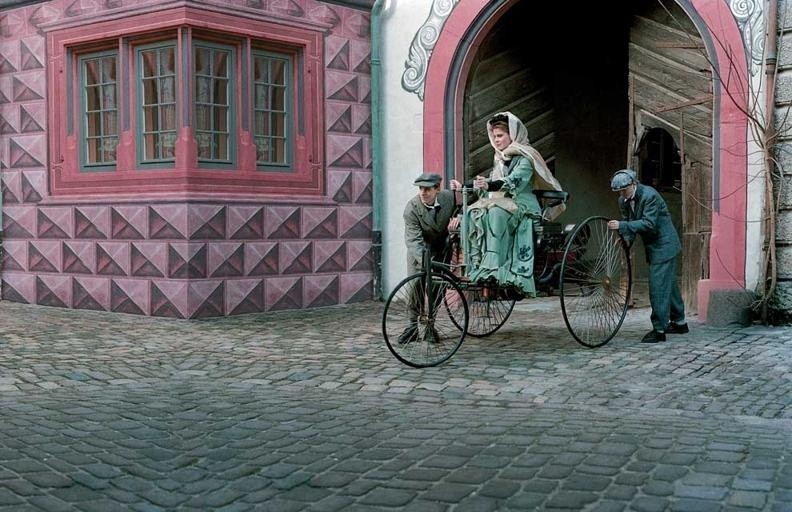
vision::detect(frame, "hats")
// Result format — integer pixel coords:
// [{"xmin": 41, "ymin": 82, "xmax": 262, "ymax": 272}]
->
[
  {"xmin": 413, "ymin": 173, "xmax": 442, "ymax": 188},
  {"xmin": 612, "ymin": 170, "xmax": 639, "ymax": 191},
  {"xmin": 489, "ymin": 114, "xmax": 525, "ymax": 132}
]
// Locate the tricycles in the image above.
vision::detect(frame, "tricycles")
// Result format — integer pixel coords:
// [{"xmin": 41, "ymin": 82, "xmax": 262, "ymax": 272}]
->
[{"xmin": 381, "ymin": 176, "xmax": 638, "ymax": 369}]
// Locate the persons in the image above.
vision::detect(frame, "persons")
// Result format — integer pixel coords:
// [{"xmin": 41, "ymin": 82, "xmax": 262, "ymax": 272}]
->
[
  {"xmin": 397, "ymin": 172, "xmax": 459, "ymax": 345},
  {"xmin": 448, "ymin": 111, "xmax": 543, "ymax": 301},
  {"xmin": 604, "ymin": 168, "xmax": 689, "ymax": 346}
]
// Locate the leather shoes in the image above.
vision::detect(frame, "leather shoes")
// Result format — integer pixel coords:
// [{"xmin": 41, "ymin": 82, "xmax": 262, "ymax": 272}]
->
[
  {"xmin": 667, "ymin": 322, "xmax": 690, "ymax": 333},
  {"xmin": 641, "ymin": 330, "xmax": 666, "ymax": 343},
  {"xmin": 398, "ymin": 327, "xmax": 419, "ymax": 344},
  {"xmin": 424, "ymin": 327, "xmax": 439, "ymax": 343}
]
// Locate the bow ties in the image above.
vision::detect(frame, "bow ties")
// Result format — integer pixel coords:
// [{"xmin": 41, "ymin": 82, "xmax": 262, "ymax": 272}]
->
[
  {"xmin": 427, "ymin": 206, "xmax": 441, "ymax": 213},
  {"xmin": 623, "ymin": 196, "xmax": 638, "ymax": 206}
]
